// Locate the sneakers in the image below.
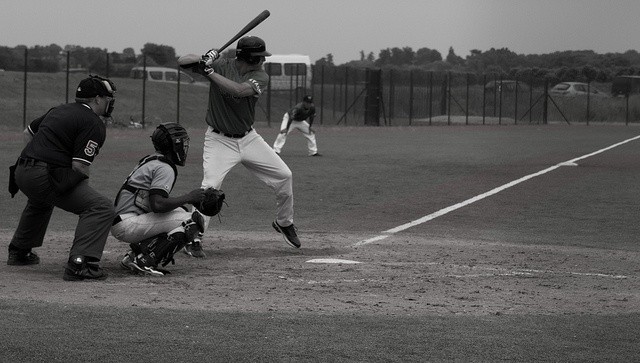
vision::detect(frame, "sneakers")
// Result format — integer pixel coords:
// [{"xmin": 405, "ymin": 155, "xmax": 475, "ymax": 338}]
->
[
  {"xmin": 272, "ymin": 219, "xmax": 301, "ymax": 248},
  {"xmin": 127, "ymin": 254, "xmax": 165, "ymax": 277},
  {"xmin": 120, "ymin": 251, "xmax": 135, "ymax": 270},
  {"xmin": 63, "ymin": 261, "xmax": 109, "ymax": 282},
  {"xmin": 7, "ymin": 251, "xmax": 39, "ymax": 265},
  {"xmin": 184, "ymin": 241, "xmax": 206, "ymax": 258}
]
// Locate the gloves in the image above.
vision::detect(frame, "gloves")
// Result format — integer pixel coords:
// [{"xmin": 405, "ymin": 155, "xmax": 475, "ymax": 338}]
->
[
  {"xmin": 205, "ymin": 49, "xmax": 220, "ymax": 62},
  {"xmin": 198, "ymin": 54, "xmax": 214, "ymax": 77}
]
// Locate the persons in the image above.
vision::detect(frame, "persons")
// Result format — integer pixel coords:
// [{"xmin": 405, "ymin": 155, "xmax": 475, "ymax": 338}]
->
[
  {"xmin": 273, "ymin": 95, "xmax": 322, "ymax": 158},
  {"xmin": 8, "ymin": 76, "xmax": 115, "ymax": 282},
  {"xmin": 110, "ymin": 122, "xmax": 225, "ymax": 278},
  {"xmin": 182, "ymin": 36, "xmax": 303, "ymax": 258}
]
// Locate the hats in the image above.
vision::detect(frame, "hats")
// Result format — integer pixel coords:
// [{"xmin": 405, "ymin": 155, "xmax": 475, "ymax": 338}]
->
[{"xmin": 304, "ymin": 95, "xmax": 313, "ymax": 104}]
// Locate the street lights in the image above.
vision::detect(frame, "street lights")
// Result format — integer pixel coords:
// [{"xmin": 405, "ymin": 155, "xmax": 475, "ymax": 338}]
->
[{"xmin": 59, "ymin": 51, "xmax": 68, "ymax": 103}]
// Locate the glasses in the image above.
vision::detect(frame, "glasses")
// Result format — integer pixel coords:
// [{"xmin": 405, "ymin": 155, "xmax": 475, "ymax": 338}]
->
[{"xmin": 246, "ymin": 56, "xmax": 265, "ymax": 66}]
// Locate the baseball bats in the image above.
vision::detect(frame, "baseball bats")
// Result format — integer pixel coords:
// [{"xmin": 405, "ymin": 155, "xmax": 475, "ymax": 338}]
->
[{"xmin": 202, "ymin": 10, "xmax": 271, "ymax": 68}]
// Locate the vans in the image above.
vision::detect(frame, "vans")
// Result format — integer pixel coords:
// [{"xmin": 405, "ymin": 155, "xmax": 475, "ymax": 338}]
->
[
  {"xmin": 132, "ymin": 66, "xmax": 197, "ymax": 84},
  {"xmin": 262, "ymin": 55, "xmax": 311, "ymax": 88},
  {"xmin": 612, "ymin": 76, "xmax": 640, "ymax": 98}
]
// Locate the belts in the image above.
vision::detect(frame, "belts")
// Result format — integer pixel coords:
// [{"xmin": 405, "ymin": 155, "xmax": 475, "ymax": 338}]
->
[
  {"xmin": 18, "ymin": 158, "xmax": 47, "ymax": 168},
  {"xmin": 112, "ymin": 215, "xmax": 122, "ymax": 229},
  {"xmin": 211, "ymin": 128, "xmax": 253, "ymax": 139}
]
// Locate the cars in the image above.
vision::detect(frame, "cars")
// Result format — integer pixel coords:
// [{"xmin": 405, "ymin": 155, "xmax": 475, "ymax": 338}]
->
[
  {"xmin": 550, "ymin": 82, "xmax": 605, "ymax": 96},
  {"xmin": 483, "ymin": 80, "xmax": 533, "ymax": 93}
]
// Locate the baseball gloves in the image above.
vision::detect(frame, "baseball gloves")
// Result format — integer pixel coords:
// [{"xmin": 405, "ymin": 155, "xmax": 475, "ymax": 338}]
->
[{"xmin": 193, "ymin": 188, "xmax": 226, "ymax": 217}]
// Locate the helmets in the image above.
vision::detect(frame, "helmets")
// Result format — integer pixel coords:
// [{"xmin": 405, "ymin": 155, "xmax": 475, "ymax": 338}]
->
[
  {"xmin": 76, "ymin": 74, "xmax": 116, "ymax": 121},
  {"xmin": 236, "ymin": 36, "xmax": 272, "ymax": 57},
  {"xmin": 152, "ymin": 122, "xmax": 190, "ymax": 166}
]
